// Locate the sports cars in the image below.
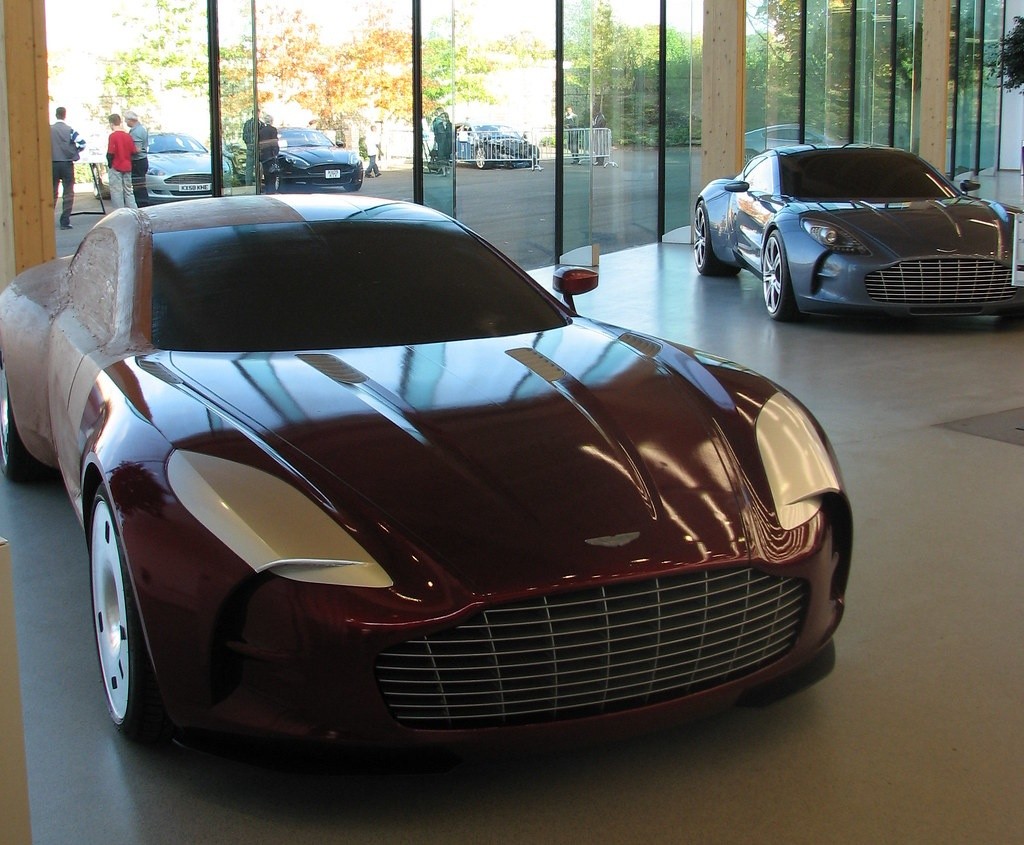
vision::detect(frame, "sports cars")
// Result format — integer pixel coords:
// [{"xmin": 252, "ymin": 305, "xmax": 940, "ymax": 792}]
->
[
  {"xmin": 693, "ymin": 138, "xmax": 1023, "ymax": 326},
  {"xmin": 0, "ymin": 185, "xmax": 856, "ymax": 754}
]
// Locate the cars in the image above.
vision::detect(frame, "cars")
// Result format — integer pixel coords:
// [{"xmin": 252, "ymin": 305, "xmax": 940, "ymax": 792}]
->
[
  {"xmin": 143, "ymin": 131, "xmax": 233, "ymax": 206},
  {"xmin": 262, "ymin": 124, "xmax": 364, "ymax": 193},
  {"xmin": 453, "ymin": 120, "xmax": 540, "ymax": 171},
  {"xmin": 743, "ymin": 123, "xmax": 832, "ymax": 170}
]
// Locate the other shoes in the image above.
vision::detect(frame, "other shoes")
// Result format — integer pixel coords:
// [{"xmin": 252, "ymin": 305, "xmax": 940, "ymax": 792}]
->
[
  {"xmin": 365, "ymin": 174, "xmax": 374, "ymax": 178},
  {"xmin": 60, "ymin": 223, "xmax": 73, "ymax": 231},
  {"xmin": 376, "ymin": 172, "xmax": 381, "ymax": 177}
]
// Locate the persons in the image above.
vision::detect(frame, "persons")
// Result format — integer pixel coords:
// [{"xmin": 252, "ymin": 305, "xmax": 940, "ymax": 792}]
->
[
  {"xmin": 49, "ymin": 107, "xmax": 85, "ymax": 229},
  {"xmin": 564, "ymin": 106, "xmax": 581, "ymax": 165},
  {"xmin": 431, "ymin": 106, "xmax": 453, "ymax": 176},
  {"xmin": 259, "ymin": 116, "xmax": 279, "ymax": 194},
  {"xmin": 123, "ymin": 110, "xmax": 149, "ymax": 208},
  {"xmin": 365, "ymin": 125, "xmax": 384, "ymax": 178},
  {"xmin": 108, "ymin": 113, "xmax": 142, "ymax": 208},
  {"xmin": 242, "ymin": 109, "xmax": 266, "ymax": 186},
  {"xmin": 592, "ymin": 104, "xmax": 606, "ymax": 166}
]
